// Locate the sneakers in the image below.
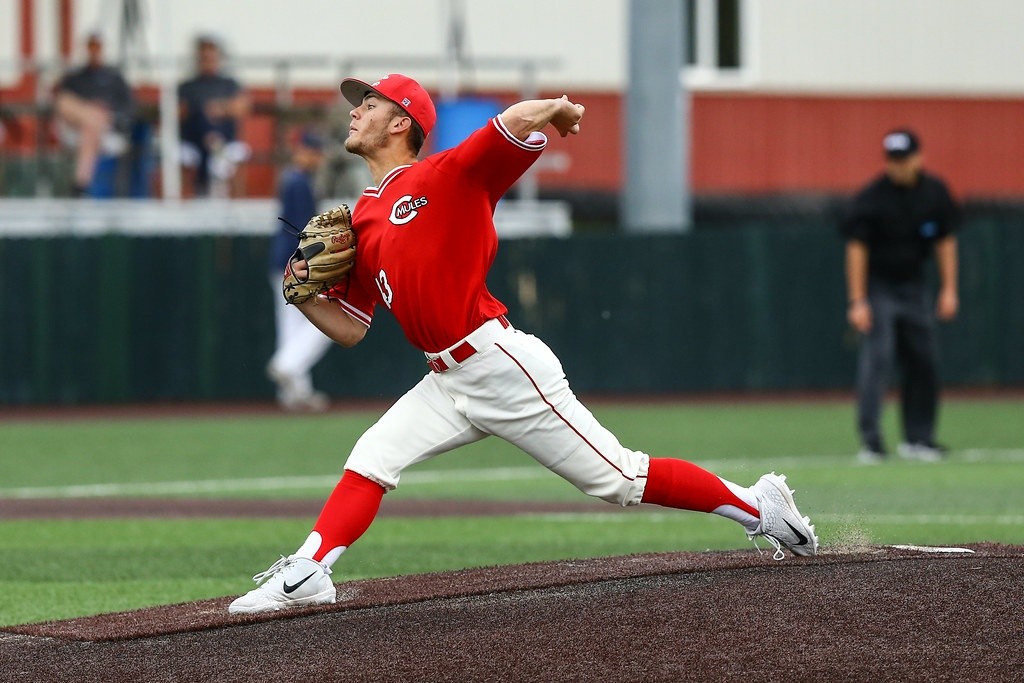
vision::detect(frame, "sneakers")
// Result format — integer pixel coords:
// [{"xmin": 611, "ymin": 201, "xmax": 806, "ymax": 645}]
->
[
  {"xmin": 744, "ymin": 471, "xmax": 819, "ymax": 560},
  {"xmin": 229, "ymin": 554, "xmax": 337, "ymax": 616}
]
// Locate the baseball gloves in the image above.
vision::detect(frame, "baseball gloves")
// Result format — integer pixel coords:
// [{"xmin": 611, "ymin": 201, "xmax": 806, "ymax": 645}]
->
[{"xmin": 275, "ymin": 202, "xmax": 357, "ymax": 307}]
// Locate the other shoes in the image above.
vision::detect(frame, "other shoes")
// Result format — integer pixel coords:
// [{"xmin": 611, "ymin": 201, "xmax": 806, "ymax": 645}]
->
[
  {"xmin": 282, "ymin": 392, "xmax": 327, "ymax": 415},
  {"xmin": 858, "ymin": 446, "xmax": 886, "ymax": 465},
  {"xmin": 898, "ymin": 439, "xmax": 946, "ymax": 460},
  {"xmin": 237, "ymin": 376, "xmax": 272, "ymax": 415}
]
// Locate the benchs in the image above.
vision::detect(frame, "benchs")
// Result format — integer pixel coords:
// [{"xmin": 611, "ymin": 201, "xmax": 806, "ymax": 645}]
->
[{"xmin": 0, "ymin": 99, "xmax": 325, "ymax": 197}]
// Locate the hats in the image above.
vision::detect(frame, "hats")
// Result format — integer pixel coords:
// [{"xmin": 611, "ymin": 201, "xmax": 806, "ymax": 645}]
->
[
  {"xmin": 340, "ymin": 73, "xmax": 436, "ymax": 140},
  {"xmin": 883, "ymin": 131, "xmax": 918, "ymax": 159},
  {"xmin": 303, "ymin": 132, "xmax": 328, "ymax": 149}
]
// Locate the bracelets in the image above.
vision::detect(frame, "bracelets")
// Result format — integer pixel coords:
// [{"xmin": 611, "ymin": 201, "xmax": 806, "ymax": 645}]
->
[{"xmin": 848, "ymin": 293, "xmax": 869, "ymax": 305}]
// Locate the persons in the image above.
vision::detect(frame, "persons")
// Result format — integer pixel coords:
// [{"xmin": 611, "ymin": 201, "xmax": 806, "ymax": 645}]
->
[
  {"xmin": 260, "ymin": 135, "xmax": 336, "ymax": 412},
  {"xmin": 226, "ymin": 73, "xmax": 818, "ymax": 613},
  {"xmin": 0, "ymin": 36, "xmax": 249, "ymax": 199},
  {"xmin": 838, "ymin": 130, "xmax": 958, "ymax": 466}
]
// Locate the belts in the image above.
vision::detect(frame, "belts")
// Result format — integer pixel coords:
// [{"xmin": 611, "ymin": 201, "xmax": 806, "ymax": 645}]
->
[{"xmin": 427, "ymin": 314, "xmax": 509, "ymax": 374}]
